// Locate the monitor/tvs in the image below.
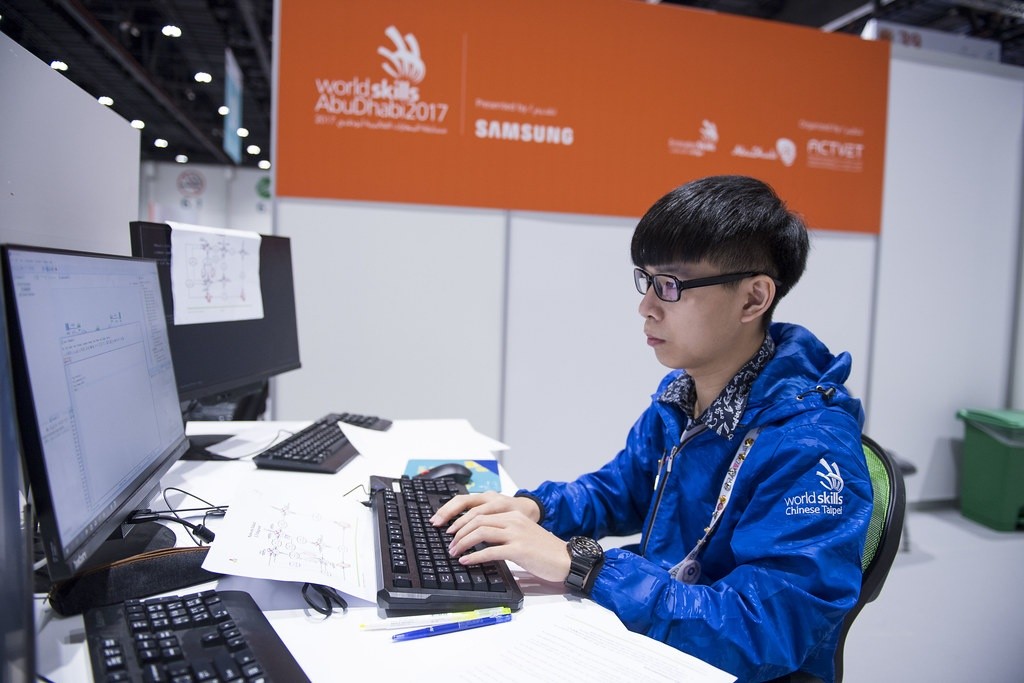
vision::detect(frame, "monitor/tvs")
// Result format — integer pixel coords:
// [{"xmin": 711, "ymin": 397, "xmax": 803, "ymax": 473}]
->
[
  {"xmin": 0, "ymin": 243, "xmax": 189, "ymax": 593},
  {"xmin": 129, "ymin": 221, "xmax": 301, "ymax": 462}
]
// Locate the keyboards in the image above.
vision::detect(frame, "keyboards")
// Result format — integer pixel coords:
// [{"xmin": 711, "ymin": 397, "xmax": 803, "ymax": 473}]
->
[
  {"xmin": 369, "ymin": 475, "xmax": 525, "ymax": 610},
  {"xmin": 83, "ymin": 589, "xmax": 311, "ymax": 683},
  {"xmin": 252, "ymin": 413, "xmax": 392, "ymax": 473}
]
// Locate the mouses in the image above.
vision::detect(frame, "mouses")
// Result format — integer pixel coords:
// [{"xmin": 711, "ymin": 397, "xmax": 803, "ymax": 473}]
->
[{"xmin": 412, "ymin": 463, "xmax": 472, "ymax": 484}]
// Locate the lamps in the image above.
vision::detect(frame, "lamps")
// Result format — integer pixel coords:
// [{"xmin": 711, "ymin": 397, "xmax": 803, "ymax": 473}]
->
[{"xmin": 130, "ymin": 5, "xmax": 183, "ymax": 37}]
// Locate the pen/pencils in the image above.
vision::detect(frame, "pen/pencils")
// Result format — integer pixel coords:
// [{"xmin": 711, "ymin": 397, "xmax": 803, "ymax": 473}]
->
[{"xmin": 362, "ymin": 607, "xmax": 511, "ymax": 639}]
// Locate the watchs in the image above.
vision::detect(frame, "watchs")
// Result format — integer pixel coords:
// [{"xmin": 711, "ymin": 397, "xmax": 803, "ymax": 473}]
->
[{"xmin": 565, "ymin": 536, "xmax": 603, "ymax": 591}]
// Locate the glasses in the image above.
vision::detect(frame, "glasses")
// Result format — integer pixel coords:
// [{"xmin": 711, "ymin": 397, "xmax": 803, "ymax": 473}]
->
[{"xmin": 633, "ymin": 268, "xmax": 784, "ymax": 302}]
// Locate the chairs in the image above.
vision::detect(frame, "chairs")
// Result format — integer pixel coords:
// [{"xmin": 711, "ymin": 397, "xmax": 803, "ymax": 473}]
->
[{"xmin": 836, "ymin": 435, "xmax": 906, "ymax": 683}]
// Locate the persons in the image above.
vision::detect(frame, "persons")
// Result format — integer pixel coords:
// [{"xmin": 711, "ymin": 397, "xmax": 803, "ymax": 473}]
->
[{"xmin": 430, "ymin": 174, "xmax": 873, "ymax": 683}]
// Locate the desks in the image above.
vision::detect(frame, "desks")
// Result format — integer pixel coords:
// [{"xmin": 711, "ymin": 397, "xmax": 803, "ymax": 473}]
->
[{"xmin": 37, "ymin": 422, "xmax": 732, "ymax": 683}]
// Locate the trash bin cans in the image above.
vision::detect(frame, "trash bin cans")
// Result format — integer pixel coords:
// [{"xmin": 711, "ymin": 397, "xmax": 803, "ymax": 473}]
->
[{"xmin": 955, "ymin": 408, "xmax": 1024, "ymax": 532}]
